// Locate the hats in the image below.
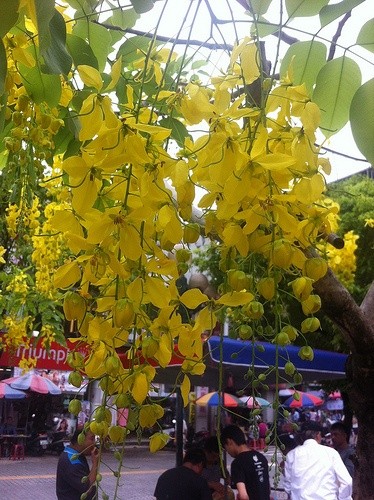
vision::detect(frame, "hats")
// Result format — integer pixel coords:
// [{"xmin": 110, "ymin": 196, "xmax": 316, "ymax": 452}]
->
[
  {"xmin": 300, "ymin": 421, "xmax": 323, "ymax": 432},
  {"xmin": 255, "ymin": 415, "xmax": 263, "ymax": 422}
]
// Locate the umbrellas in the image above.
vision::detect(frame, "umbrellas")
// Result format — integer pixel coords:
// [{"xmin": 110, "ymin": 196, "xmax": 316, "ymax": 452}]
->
[
  {"xmin": 0, "ymin": 383, "xmax": 26, "ymax": 400},
  {"xmin": 1, "ymin": 373, "xmax": 62, "ymax": 396}
]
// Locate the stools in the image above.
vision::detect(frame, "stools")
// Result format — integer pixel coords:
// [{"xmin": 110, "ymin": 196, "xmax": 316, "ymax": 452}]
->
[{"xmin": 9, "ymin": 444, "xmax": 24, "ymax": 461}]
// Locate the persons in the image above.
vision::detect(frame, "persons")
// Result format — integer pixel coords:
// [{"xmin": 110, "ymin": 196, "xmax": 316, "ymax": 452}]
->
[
  {"xmin": 153, "ymin": 447, "xmax": 215, "ymax": 500},
  {"xmin": 330, "ymin": 422, "xmax": 356, "ymax": 479},
  {"xmin": 198, "ymin": 437, "xmax": 235, "ymax": 500},
  {"xmin": 220, "ymin": 424, "xmax": 271, "ymax": 500},
  {"xmin": 0, "ymin": 417, "xmax": 17, "ymax": 457},
  {"xmin": 56, "ymin": 425, "xmax": 100, "ymax": 500},
  {"xmin": 281, "ymin": 419, "xmax": 353, "ymax": 500},
  {"xmin": 249, "ymin": 409, "xmax": 358, "ymax": 451}
]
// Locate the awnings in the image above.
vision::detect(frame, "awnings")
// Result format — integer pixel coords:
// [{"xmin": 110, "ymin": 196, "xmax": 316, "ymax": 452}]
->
[{"xmin": 117, "ymin": 336, "xmax": 348, "ymax": 380}]
